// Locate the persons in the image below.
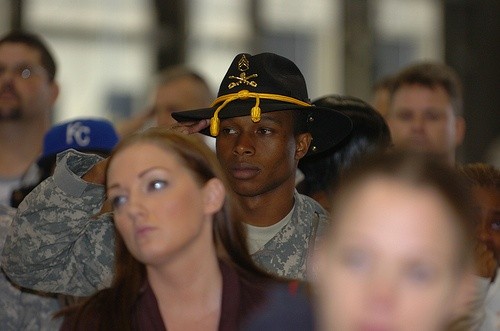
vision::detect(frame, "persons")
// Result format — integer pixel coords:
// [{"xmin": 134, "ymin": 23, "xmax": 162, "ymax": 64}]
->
[
  {"xmin": 0, "ymin": 30, "xmax": 500, "ymax": 331},
  {"xmin": 0, "ymin": 53, "xmax": 327, "ymax": 297}
]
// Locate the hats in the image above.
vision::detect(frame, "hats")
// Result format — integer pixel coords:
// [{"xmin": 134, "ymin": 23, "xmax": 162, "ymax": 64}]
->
[
  {"xmin": 43, "ymin": 119, "xmax": 119, "ymax": 157},
  {"xmin": 171, "ymin": 52, "xmax": 354, "ymax": 158}
]
